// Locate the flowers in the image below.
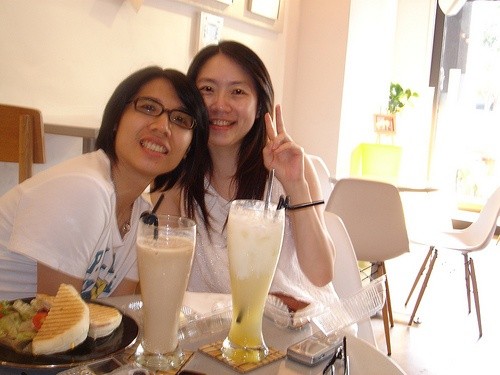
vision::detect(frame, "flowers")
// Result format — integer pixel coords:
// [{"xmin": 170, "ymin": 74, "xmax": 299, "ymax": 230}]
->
[{"xmin": 387, "ymin": 83, "xmax": 423, "ymax": 115}]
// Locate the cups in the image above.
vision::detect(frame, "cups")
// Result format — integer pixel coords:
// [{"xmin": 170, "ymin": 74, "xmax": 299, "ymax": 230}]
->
[
  {"xmin": 221, "ymin": 199, "xmax": 285, "ymax": 364},
  {"xmin": 134, "ymin": 214, "xmax": 196, "ymax": 371}
]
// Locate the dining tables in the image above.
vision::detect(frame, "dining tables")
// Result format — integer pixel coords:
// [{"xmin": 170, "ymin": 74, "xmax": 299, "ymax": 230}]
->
[{"xmin": 92, "ymin": 293, "xmax": 407, "ymax": 375}]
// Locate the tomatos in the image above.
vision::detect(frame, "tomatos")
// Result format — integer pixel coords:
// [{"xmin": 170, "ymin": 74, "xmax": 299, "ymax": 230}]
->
[{"xmin": 33, "ymin": 310, "xmax": 48, "ymax": 329}]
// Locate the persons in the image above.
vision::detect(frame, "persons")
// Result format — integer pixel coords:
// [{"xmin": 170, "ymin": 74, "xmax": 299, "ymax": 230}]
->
[
  {"xmin": 0, "ymin": 66, "xmax": 213, "ymax": 298},
  {"xmin": 144, "ymin": 39, "xmax": 358, "ymax": 339}
]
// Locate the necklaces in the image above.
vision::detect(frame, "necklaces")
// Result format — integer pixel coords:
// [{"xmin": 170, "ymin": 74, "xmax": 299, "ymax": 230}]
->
[{"xmin": 114, "ymin": 186, "xmax": 131, "ymax": 233}]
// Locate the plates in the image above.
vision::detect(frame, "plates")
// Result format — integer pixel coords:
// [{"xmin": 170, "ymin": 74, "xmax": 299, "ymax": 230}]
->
[{"xmin": 0, "ymin": 296, "xmax": 141, "ymax": 374}]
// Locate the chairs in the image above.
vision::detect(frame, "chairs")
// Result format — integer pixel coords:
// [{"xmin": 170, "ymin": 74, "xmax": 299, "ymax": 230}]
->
[
  {"xmin": 0, "ymin": 105, "xmax": 46, "ymax": 184},
  {"xmin": 401, "ymin": 186, "xmax": 500, "ymax": 338},
  {"xmin": 323, "ymin": 178, "xmax": 407, "ymax": 356}
]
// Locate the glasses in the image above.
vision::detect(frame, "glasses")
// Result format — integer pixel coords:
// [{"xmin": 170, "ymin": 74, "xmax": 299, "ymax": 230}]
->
[{"xmin": 128, "ymin": 95, "xmax": 197, "ymax": 130}]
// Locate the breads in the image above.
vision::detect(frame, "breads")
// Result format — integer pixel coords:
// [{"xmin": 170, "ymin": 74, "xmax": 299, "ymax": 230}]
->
[
  {"xmin": 87, "ymin": 303, "xmax": 122, "ymax": 339},
  {"xmin": 31, "ymin": 283, "xmax": 89, "ymax": 354}
]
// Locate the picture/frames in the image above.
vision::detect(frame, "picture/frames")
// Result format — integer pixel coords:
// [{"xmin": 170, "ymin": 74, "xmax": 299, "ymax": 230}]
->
[{"xmin": 373, "ymin": 114, "xmax": 396, "ymax": 135}]
